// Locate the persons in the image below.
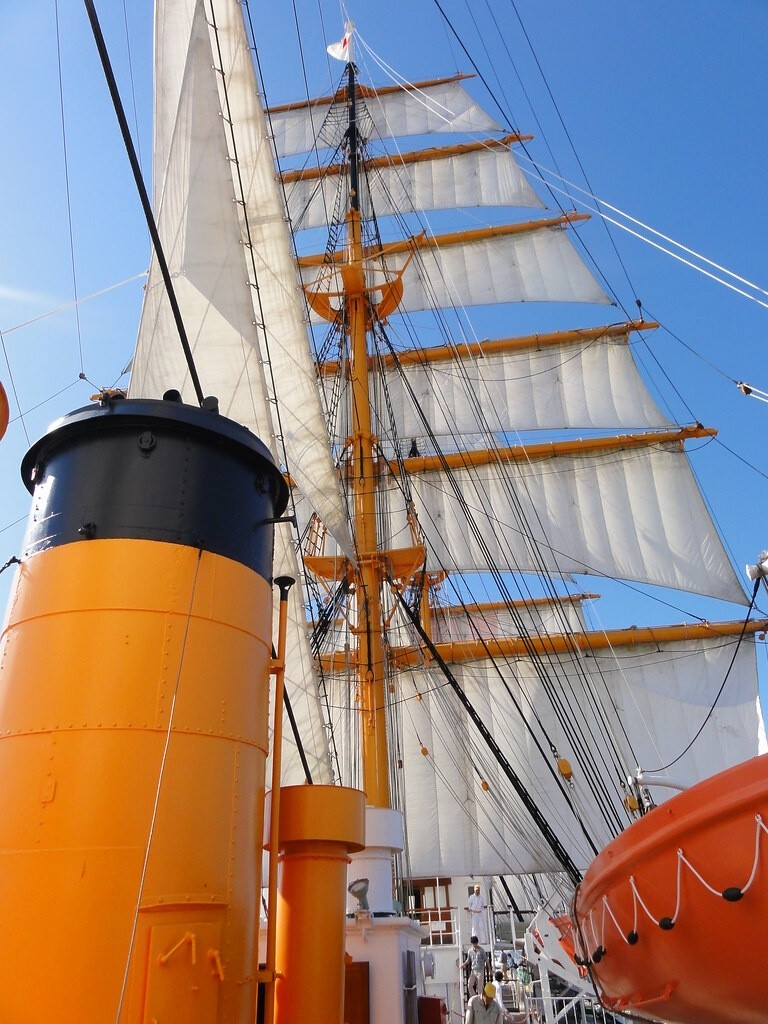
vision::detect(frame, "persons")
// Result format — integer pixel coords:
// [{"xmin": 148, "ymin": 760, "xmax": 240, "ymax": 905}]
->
[
  {"xmin": 498, "ymin": 950, "xmax": 507, "ymax": 980},
  {"xmin": 507, "ymin": 953, "xmax": 518, "ymax": 981},
  {"xmin": 464, "ymin": 884, "xmax": 490, "ymax": 945},
  {"xmin": 460, "ymin": 936, "xmax": 492, "ymax": 999},
  {"xmin": 464, "ymin": 983, "xmax": 504, "ymax": 1024},
  {"xmin": 492, "ymin": 971, "xmax": 514, "ymax": 1024}
]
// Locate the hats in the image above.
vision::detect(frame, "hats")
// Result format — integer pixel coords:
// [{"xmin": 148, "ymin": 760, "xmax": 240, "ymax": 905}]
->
[{"xmin": 484, "ymin": 982, "xmax": 496, "ymax": 998}]
